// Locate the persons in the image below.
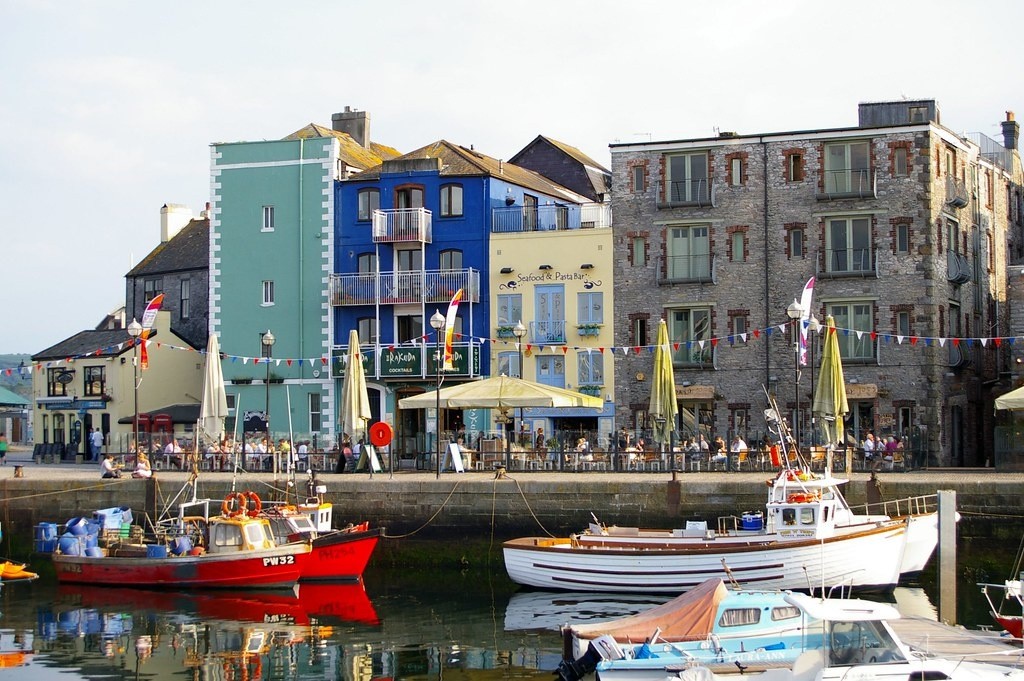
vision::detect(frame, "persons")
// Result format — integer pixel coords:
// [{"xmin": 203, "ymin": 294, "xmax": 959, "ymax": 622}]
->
[
  {"xmin": 89, "ymin": 427, "xmax": 104, "ymax": 462},
  {"xmin": 100, "ymin": 455, "xmax": 124, "ymax": 478},
  {"xmin": 129, "ymin": 431, "xmax": 365, "ymax": 471},
  {"xmin": 132, "ymin": 453, "xmax": 152, "ymax": 479},
  {"xmin": 453, "ymin": 425, "xmax": 904, "ymax": 470}
]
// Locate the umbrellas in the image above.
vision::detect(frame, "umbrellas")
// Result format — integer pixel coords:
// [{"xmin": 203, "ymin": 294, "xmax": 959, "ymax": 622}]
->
[
  {"xmin": 337, "ymin": 328, "xmax": 372, "ymax": 450},
  {"xmin": 648, "ymin": 319, "xmax": 678, "ymax": 471},
  {"xmin": 397, "ymin": 373, "xmax": 605, "ymax": 470},
  {"xmin": 994, "ymin": 383, "xmax": 1024, "ymax": 417},
  {"xmin": 811, "ymin": 313, "xmax": 850, "ymax": 470},
  {"xmin": 199, "ymin": 333, "xmax": 229, "ymax": 443}
]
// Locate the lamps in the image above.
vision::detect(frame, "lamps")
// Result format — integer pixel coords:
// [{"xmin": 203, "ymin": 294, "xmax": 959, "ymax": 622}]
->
[
  {"xmin": 500, "ymin": 268, "xmax": 514, "ymax": 273},
  {"xmin": 539, "ymin": 265, "xmax": 553, "ymax": 269},
  {"xmin": 581, "ymin": 264, "xmax": 594, "ymax": 269}
]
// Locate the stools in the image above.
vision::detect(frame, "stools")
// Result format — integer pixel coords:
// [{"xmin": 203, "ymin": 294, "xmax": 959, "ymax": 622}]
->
[
  {"xmin": 155, "ymin": 460, "xmax": 348, "ymax": 471},
  {"xmin": 452, "ymin": 460, "xmax": 848, "ymax": 470}
]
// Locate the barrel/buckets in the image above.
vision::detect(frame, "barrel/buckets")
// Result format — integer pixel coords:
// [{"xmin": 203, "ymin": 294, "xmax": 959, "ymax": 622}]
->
[
  {"xmin": 37, "ymin": 517, "xmax": 103, "ymax": 558},
  {"xmin": 119, "ymin": 522, "xmax": 130, "ymax": 538},
  {"xmin": 146, "ymin": 544, "xmax": 166, "ymax": 558},
  {"xmin": 742, "ymin": 514, "xmax": 762, "ymax": 531}
]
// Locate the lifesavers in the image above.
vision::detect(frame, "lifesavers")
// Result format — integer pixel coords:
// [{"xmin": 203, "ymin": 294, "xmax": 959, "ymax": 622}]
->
[
  {"xmin": 778, "ymin": 471, "xmax": 804, "ymax": 480},
  {"xmin": 788, "ymin": 493, "xmax": 815, "ymax": 503},
  {"xmin": 222, "ymin": 492, "xmax": 247, "ymax": 517},
  {"xmin": 239, "ymin": 491, "xmax": 261, "ymax": 517},
  {"xmin": 224, "ymin": 657, "xmax": 262, "ymax": 681}
]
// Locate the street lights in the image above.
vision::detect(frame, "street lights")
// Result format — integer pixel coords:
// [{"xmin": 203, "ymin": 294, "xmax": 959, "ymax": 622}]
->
[
  {"xmin": 808, "ymin": 312, "xmax": 819, "ymax": 447},
  {"xmin": 261, "ymin": 328, "xmax": 276, "ymax": 453},
  {"xmin": 786, "ymin": 297, "xmax": 804, "ymax": 449},
  {"xmin": 512, "ymin": 317, "xmax": 527, "ymax": 445},
  {"xmin": 429, "ymin": 308, "xmax": 446, "ymax": 477}
]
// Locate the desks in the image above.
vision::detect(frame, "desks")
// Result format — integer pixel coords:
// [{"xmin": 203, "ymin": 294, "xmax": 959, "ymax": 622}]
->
[
  {"xmin": 718, "ymin": 449, "xmax": 740, "ymax": 471},
  {"xmin": 260, "ymin": 451, "xmax": 282, "ymax": 474},
  {"xmin": 558, "ymin": 452, "xmax": 579, "ymax": 472},
  {"xmin": 308, "ymin": 453, "xmax": 326, "ymax": 471},
  {"xmin": 213, "ymin": 452, "xmax": 232, "ymax": 470},
  {"xmin": 664, "ymin": 451, "xmax": 686, "ymax": 471},
  {"xmin": 451, "ymin": 450, "xmax": 477, "ymax": 471},
  {"xmin": 611, "ymin": 452, "xmax": 630, "ymax": 471},
  {"xmin": 167, "ymin": 453, "xmax": 186, "ymax": 470},
  {"xmin": 506, "ymin": 452, "xmax": 526, "ymax": 472}
]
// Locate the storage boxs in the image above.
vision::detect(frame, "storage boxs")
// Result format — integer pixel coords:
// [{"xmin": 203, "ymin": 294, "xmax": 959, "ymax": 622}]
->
[
  {"xmin": 93, "ymin": 509, "xmax": 123, "ymax": 529},
  {"xmin": 743, "ymin": 513, "xmax": 762, "ymax": 531}
]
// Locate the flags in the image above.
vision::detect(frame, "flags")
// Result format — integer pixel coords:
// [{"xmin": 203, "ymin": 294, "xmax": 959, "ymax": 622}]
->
[{"xmin": 798, "ymin": 276, "xmax": 815, "ymax": 366}]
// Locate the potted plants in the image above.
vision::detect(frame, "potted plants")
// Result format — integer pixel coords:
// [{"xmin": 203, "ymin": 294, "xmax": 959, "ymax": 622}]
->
[
  {"xmin": 577, "ymin": 324, "xmax": 600, "ymax": 336},
  {"xmin": 692, "ymin": 352, "xmax": 704, "ymax": 363},
  {"xmin": 580, "ymin": 384, "xmax": 600, "ymax": 397},
  {"xmin": 496, "ymin": 326, "xmax": 518, "ymax": 338}
]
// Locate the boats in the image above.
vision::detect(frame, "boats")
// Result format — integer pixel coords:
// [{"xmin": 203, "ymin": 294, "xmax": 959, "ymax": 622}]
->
[
  {"xmin": 974, "ymin": 577, "xmax": 1023, "ymax": 647},
  {"xmin": 503, "ymin": 468, "xmax": 962, "ymax": 594},
  {"xmin": 34, "ymin": 493, "xmax": 312, "ymax": 590},
  {"xmin": 246, "ymin": 486, "xmax": 385, "ymax": 584},
  {"xmin": 557, "ymin": 576, "xmax": 1020, "ymax": 681}
]
